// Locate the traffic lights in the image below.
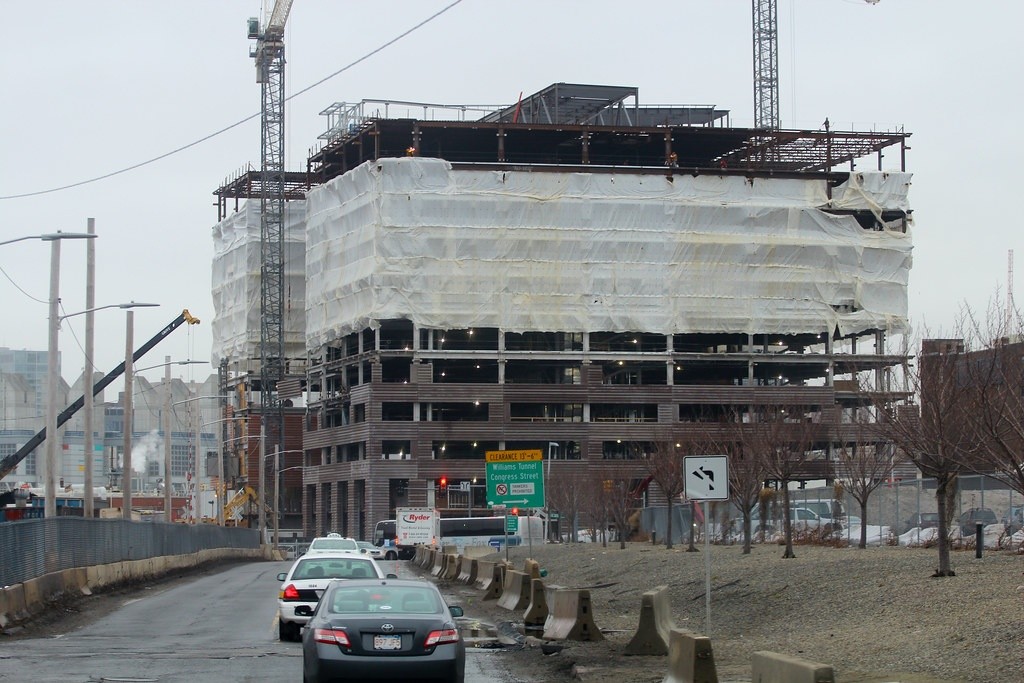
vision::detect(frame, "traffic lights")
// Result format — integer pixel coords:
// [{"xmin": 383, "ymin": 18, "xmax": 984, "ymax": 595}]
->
[
  {"xmin": 441, "ymin": 479, "xmax": 446, "ymax": 489},
  {"xmin": 512, "ymin": 508, "xmax": 519, "ymax": 515}
]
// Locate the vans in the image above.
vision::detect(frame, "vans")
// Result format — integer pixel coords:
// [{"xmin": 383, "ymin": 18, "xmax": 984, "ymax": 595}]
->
[{"xmin": 742, "ymin": 499, "xmax": 847, "ymax": 528}]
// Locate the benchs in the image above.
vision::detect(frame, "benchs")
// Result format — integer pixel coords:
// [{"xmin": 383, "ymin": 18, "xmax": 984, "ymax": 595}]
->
[{"xmin": 338, "ymin": 601, "xmax": 433, "ymax": 611}]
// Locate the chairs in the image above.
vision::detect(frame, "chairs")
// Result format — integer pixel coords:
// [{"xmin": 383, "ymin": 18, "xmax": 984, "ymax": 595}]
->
[
  {"xmin": 352, "ymin": 568, "xmax": 367, "ymax": 577},
  {"xmin": 307, "ymin": 569, "xmax": 323, "ymax": 577},
  {"xmin": 402, "ymin": 593, "xmax": 425, "ymax": 609}
]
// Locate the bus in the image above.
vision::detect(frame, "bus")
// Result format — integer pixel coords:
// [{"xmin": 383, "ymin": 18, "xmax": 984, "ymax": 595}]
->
[{"xmin": 373, "ymin": 517, "xmax": 543, "ymax": 560}]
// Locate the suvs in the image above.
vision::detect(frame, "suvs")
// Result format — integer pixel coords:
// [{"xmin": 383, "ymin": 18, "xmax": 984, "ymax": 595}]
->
[{"xmin": 955, "ymin": 508, "xmax": 998, "ymax": 536}]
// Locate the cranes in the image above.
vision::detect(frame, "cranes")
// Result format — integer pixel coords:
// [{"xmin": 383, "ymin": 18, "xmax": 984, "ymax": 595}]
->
[
  {"xmin": 247, "ymin": 0, "xmax": 295, "ymax": 523},
  {"xmin": 751, "ymin": 0, "xmax": 878, "ymax": 161}
]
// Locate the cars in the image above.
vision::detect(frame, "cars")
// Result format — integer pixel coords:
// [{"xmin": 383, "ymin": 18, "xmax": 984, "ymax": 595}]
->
[
  {"xmin": 356, "ymin": 541, "xmax": 386, "ymax": 560},
  {"xmin": 742, "ymin": 508, "xmax": 843, "ymax": 535},
  {"xmin": 903, "ymin": 513, "xmax": 940, "ymax": 533},
  {"xmin": 1001, "ymin": 504, "xmax": 1024, "ymax": 524},
  {"xmin": 300, "ymin": 577, "xmax": 465, "ymax": 683},
  {"xmin": 299, "ymin": 537, "xmax": 362, "ymax": 555},
  {"xmin": 847, "ymin": 516, "xmax": 861, "ymax": 524},
  {"xmin": 277, "ymin": 554, "xmax": 398, "ymax": 644}
]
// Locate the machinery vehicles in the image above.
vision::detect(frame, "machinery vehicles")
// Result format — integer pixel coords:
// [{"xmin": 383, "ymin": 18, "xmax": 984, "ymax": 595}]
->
[
  {"xmin": 0, "ymin": 309, "xmax": 202, "ymax": 481},
  {"xmin": 175, "ymin": 485, "xmax": 274, "ymax": 528}
]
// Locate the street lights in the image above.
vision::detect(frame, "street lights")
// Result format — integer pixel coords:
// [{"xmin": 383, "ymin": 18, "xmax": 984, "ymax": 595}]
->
[
  {"xmin": 274, "ymin": 466, "xmax": 312, "ymax": 546},
  {"xmin": 47, "ymin": 302, "xmax": 161, "ymax": 519},
  {"xmin": 123, "ymin": 360, "xmax": 210, "ymax": 518},
  {"xmin": 258, "ymin": 450, "xmax": 304, "ymax": 546},
  {"xmin": 218, "ymin": 435, "xmax": 266, "ymax": 526},
  {"xmin": 195, "ymin": 418, "xmax": 250, "ymax": 523},
  {"xmin": 544, "ymin": 442, "xmax": 558, "ymax": 542},
  {"xmin": 162, "ymin": 396, "xmax": 235, "ymax": 520}
]
